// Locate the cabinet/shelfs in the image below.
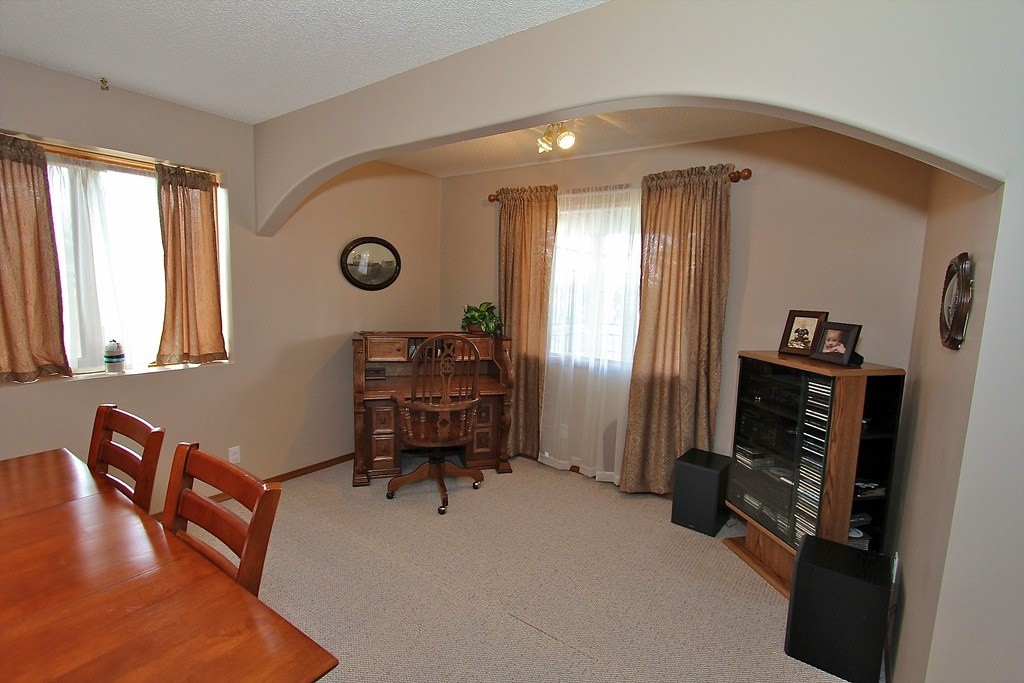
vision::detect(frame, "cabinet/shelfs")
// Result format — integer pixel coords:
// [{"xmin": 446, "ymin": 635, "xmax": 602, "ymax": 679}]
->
[
  {"xmin": 351, "ymin": 330, "xmax": 515, "ymax": 487},
  {"xmin": 723, "ymin": 350, "xmax": 907, "ymax": 601}
]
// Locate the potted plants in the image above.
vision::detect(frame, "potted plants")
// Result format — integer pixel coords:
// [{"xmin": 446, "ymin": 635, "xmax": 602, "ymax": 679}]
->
[{"xmin": 461, "ymin": 302, "xmax": 503, "ymax": 334}]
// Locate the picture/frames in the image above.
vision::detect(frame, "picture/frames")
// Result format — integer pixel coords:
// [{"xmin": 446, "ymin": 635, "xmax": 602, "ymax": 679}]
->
[
  {"xmin": 809, "ymin": 321, "xmax": 864, "ymax": 367},
  {"xmin": 778, "ymin": 310, "xmax": 829, "ymax": 356},
  {"xmin": 340, "ymin": 236, "xmax": 402, "ymax": 291}
]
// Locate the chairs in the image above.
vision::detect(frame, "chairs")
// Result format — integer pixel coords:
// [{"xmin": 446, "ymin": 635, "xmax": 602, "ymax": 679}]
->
[
  {"xmin": 386, "ymin": 334, "xmax": 484, "ymax": 515},
  {"xmin": 163, "ymin": 441, "xmax": 282, "ymax": 598},
  {"xmin": 87, "ymin": 403, "xmax": 165, "ymax": 515}
]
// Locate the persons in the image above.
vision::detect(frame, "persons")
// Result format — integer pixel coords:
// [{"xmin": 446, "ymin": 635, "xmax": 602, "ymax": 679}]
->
[
  {"xmin": 822, "ymin": 330, "xmax": 846, "ymax": 353},
  {"xmin": 789, "ymin": 319, "xmax": 811, "ymax": 347}
]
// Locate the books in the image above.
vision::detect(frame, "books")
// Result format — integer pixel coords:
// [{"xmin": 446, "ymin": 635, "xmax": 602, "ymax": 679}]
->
[
  {"xmin": 734, "ymin": 376, "xmax": 832, "ymax": 551},
  {"xmin": 855, "ymin": 474, "xmax": 886, "ymax": 498},
  {"xmin": 847, "ymin": 513, "xmax": 872, "ymax": 551}
]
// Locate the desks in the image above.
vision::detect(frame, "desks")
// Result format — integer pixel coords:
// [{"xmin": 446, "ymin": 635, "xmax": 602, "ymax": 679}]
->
[{"xmin": 0, "ymin": 447, "xmax": 338, "ymax": 683}]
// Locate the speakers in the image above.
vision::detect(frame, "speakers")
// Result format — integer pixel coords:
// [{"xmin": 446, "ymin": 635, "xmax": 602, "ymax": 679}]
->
[
  {"xmin": 671, "ymin": 447, "xmax": 732, "ymax": 537},
  {"xmin": 783, "ymin": 535, "xmax": 892, "ymax": 683}
]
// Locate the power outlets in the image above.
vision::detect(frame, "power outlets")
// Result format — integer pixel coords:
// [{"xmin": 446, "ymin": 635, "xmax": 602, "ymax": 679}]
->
[{"xmin": 229, "ymin": 446, "xmax": 240, "ymax": 464}]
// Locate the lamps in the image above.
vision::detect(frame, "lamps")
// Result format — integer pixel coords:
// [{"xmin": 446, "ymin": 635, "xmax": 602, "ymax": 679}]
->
[{"xmin": 536, "ymin": 119, "xmax": 575, "ymax": 153}]
